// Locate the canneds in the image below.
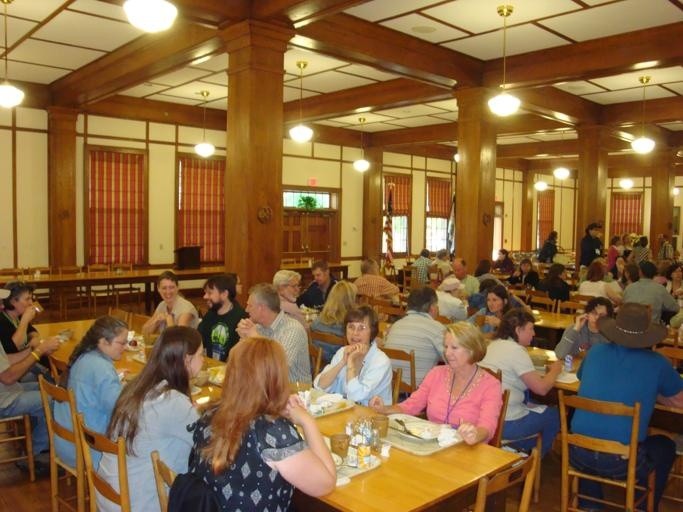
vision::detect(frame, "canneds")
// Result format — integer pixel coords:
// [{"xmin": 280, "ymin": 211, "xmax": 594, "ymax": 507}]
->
[
  {"xmin": 564, "ymin": 354, "xmax": 573, "ymax": 373},
  {"xmin": 356, "ymin": 443, "xmax": 372, "ymax": 468}
]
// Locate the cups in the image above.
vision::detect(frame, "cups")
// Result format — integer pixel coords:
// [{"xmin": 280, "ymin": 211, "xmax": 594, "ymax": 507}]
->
[
  {"xmin": 330, "ymin": 434, "xmax": 350, "ymax": 458},
  {"xmin": 477, "ymin": 314, "xmax": 485, "ymax": 326},
  {"xmin": 372, "ymin": 414, "xmax": 391, "ymax": 437},
  {"xmin": 31, "ymin": 301, "xmax": 44, "ymax": 317}
]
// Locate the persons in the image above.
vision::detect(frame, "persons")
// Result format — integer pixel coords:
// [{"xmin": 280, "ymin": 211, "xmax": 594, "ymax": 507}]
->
[{"xmin": 0, "ymin": 221, "xmax": 683, "ymax": 512}]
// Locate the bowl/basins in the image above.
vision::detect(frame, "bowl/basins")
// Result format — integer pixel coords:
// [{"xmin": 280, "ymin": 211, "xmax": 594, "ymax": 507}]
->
[
  {"xmin": 330, "ymin": 451, "xmax": 343, "ymax": 469},
  {"xmin": 195, "ymin": 371, "xmax": 211, "ymax": 387}
]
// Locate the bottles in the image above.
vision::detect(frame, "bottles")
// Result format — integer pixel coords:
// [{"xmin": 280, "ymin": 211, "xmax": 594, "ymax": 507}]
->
[
  {"xmin": 564, "ymin": 352, "xmax": 573, "ymax": 373},
  {"xmin": 345, "ymin": 417, "xmax": 381, "ymax": 471}
]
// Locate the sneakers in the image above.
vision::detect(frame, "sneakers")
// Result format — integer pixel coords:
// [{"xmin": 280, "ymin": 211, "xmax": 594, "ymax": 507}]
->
[{"xmin": 16, "ymin": 452, "xmax": 66, "ymax": 478}]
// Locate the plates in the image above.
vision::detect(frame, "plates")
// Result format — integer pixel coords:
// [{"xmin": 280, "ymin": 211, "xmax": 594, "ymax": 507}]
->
[
  {"xmin": 399, "ymin": 422, "xmax": 446, "ymax": 443},
  {"xmin": 125, "ymin": 336, "xmax": 152, "ymax": 352}
]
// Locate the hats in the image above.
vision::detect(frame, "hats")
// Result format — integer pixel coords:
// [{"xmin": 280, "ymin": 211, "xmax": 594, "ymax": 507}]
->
[
  {"xmin": 588, "ymin": 223, "xmax": 601, "ymax": 230},
  {"xmin": 595, "ymin": 303, "xmax": 668, "ymax": 348},
  {"xmin": 442, "ymin": 278, "xmax": 466, "ymax": 291}
]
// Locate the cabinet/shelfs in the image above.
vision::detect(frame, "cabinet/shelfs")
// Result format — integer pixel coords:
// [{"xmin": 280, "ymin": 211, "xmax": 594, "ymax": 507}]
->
[{"xmin": 284, "ymin": 207, "xmax": 341, "ymax": 285}]
